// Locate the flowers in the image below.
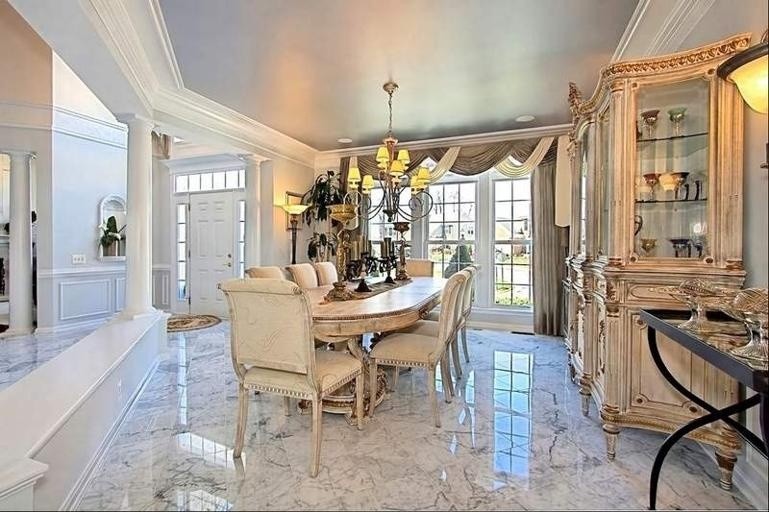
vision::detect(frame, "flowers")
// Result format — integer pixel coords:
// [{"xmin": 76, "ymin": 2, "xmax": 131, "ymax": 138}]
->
[{"xmin": 95, "ymin": 215, "xmax": 126, "ymax": 251}]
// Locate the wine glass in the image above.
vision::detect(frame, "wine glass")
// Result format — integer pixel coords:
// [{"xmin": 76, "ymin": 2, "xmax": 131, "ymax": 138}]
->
[
  {"xmin": 667, "ymin": 107, "xmax": 687, "ymax": 137},
  {"xmin": 643, "ymin": 173, "xmax": 661, "ymax": 202},
  {"xmin": 640, "ymin": 238, "xmax": 657, "ymax": 258},
  {"xmin": 691, "ymin": 235, "xmax": 706, "ymax": 258},
  {"xmin": 641, "ymin": 109, "xmax": 660, "ymax": 140},
  {"xmin": 671, "ymin": 172, "xmax": 689, "ymax": 199}
]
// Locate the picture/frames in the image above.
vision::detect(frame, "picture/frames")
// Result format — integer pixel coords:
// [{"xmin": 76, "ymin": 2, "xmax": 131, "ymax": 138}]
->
[{"xmin": 285, "ymin": 192, "xmax": 304, "ymax": 230}]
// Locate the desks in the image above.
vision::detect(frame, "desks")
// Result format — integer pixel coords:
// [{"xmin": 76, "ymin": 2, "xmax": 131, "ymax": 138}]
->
[{"xmin": 639, "ymin": 308, "xmax": 769, "ymax": 511}]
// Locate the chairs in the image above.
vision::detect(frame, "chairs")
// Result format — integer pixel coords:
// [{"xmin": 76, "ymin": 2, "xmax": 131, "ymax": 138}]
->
[
  {"xmin": 216, "ymin": 278, "xmax": 363, "ymax": 477},
  {"xmin": 368, "ymin": 271, "xmax": 470, "ymax": 427},
  {"xmin": 285, "ymin": 263, "xmax": 318, "ymax": 289},
  {"xmin": 312, "ymin": 261, "xmax": 338, "ymax": 286},
  {"xmin": 245, "ymin": 266, "xmax": 328, "ymax": 349},
  {"xmin": 423, "ymin": 264, "xmax": 482, "ymax": 373},
  {"xmin": 395, "ymin": 267, "xmax": 476, "ymax": 378},
  {"xmin": 406, "ymin": 259, "xmax": 434, "ymax": 277}
]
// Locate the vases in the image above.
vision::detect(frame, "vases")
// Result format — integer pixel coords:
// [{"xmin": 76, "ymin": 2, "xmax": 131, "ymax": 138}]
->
[{"xmin": 104, "ymin": 240, "xmax": 118, "ymax": 256}]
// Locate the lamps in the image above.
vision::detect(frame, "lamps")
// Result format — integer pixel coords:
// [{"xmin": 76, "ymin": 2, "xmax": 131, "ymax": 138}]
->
[
  {"xmin": 282, "ymin": 205, "xmax": 310, "ymax": 266},
  {"xmin": 717, "ymin": 29, "xmax": 769, "ymax": 170},
  {"xmin": 347, "ymin": 83, "xmax": 433, "ymax": 221}
]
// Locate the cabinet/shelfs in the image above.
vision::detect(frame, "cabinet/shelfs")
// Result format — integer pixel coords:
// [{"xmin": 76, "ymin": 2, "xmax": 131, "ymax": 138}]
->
[
  {"xmin": 593, "ymin": 33, "xmax": 751, "ymax": 491},
  {"xmin": 561, "ymin": 81, "xmax": 593, "ymax": 416}
]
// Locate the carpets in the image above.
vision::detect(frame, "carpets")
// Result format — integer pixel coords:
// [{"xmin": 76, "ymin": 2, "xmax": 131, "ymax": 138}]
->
[{"xmin": 166, "ymin": 314, "xmax": 221, "ymax": 332}]
